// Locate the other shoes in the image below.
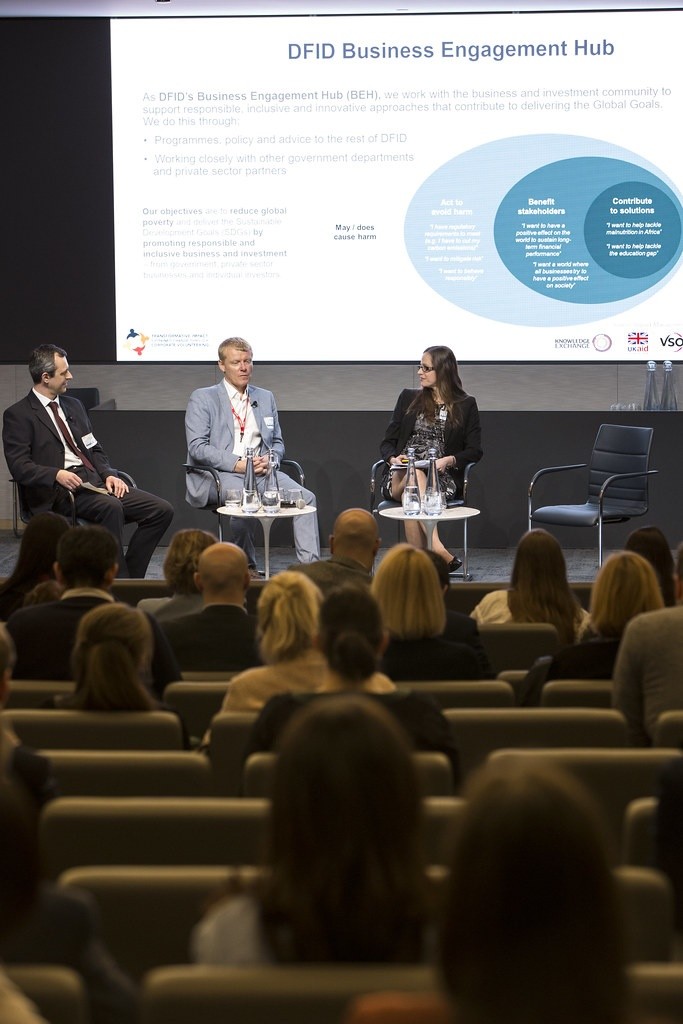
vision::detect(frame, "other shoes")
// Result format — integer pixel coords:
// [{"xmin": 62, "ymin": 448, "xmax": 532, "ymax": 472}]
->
[{"xmin": 248, "ymin": 568, "xmax": 264, "ymax": 580}]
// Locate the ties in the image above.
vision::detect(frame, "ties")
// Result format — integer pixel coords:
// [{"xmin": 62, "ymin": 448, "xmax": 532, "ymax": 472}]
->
[{"xmin": 48, "ymin": 401, "xmax": 96, "ymax": 473}]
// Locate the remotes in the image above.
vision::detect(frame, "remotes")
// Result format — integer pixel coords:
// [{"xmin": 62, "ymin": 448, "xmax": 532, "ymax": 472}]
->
[{"xmin": 402, "ymin": 459, "xmax": 408, "ymax": 464}]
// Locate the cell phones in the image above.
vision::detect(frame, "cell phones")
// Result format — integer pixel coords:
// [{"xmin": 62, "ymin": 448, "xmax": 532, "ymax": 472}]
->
[{"xmin": 280, "ymin": 500, "xmax": 297, "ymax": 508}]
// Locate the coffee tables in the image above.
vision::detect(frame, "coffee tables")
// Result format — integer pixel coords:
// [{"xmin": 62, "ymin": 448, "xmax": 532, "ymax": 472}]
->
[
  {"xmin": 379, "ymin": 506, "xmax": 481, "ymax": 580},
  {"xmin": 217, "ymin": 505, "xmax": 317, "ymax": 580}
]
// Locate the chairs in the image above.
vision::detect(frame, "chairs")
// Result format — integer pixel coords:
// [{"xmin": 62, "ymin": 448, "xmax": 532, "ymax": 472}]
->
[
  {"xmin": 368, "ymin": 460, "xmax": 475, "ymax": 550},
  {"xmin": 0, "ymin": 569, "xmax": 683, "ymax": 1024},
  {"xmin": 183, "ymin": 460, "xmax": 307, "ymax": 544},
  {"xmin": 9, "ymin": 470, "xmax": 137, "ymax": 539},
  {"xmin": 528, "ymin": 423, "xmax": 659, "ymax": 566}
]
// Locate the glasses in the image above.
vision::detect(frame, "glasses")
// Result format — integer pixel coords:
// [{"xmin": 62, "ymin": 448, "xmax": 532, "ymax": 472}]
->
[{"xmin": 417, "ymin": 365, "xmax": 434, "ymax": 372}]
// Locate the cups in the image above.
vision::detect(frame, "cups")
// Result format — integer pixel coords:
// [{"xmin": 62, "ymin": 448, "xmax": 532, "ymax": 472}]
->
[
  {"xmin": 271, "ymin": 487, "xmax": 303, "ymax": 503},
  {"xmin": 424, "ymin": 487, "xmax": 442, "ymax": 515},
  {"xmin": 262, "ymin": 491, "xmax": 281, "ymax": 514},
  {"xmin": 402, "ymin": 486, "xmax": 421, "ymax": 515},
  {"xmin": 225, "ymin": 489, "xmax": 243, "ymax": 509},
  {"xmin": 242, "ymin": 490, "xmax": 262, "ymax": 514}
]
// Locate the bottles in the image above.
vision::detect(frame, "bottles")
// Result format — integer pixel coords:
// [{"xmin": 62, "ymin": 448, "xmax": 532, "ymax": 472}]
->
[
  {"xmin": 660, "ymin": 361, "xmax": 677, "ymax": 410},
  {"xmin": 643, "ymin": 361, "xmax": 661, "ymax": 410},
  {"xmin": 426, "ymin": 448, "xmax": 441, "ymax": 492},
  {"xmin": 264, "ymin": 449, "xmax": 280, "ymax": 492},
  {"xmin": 243, "ymin": 448, "xmax": 258, "ymax": 493},
  {"xmin": 405, "ymin": 447, "xmax": 418, "ymax": 487}
]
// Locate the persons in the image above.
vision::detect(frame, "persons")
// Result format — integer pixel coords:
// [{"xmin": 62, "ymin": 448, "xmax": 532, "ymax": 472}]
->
[
  {"xmin": 184, "ymin": 336, "xmax": 321, "ymax": 572},
  {"xmin": 379, "ymin": 345, "xmax": 485, "ymax": 574},
  {"xmin": 2, "ymin": 343, "xmax": 176, "ymax": 579},
  {"xmin": 0, "ymin": 507, "xmax": 683, "ymax": 1024}
]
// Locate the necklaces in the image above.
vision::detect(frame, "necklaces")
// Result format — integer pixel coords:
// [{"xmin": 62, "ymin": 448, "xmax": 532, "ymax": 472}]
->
[{"xmin": 231, "ymin": 394, "xmax": 249, "ymax": 443}]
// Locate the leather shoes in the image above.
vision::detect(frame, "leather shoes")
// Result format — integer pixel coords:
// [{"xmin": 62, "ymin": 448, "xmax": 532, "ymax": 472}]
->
[{"xmin": 447, "ymin": 556, "xmax": 463, "ymax": 573}]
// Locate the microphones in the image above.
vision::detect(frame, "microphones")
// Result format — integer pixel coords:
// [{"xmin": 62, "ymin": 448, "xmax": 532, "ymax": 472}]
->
[
  {"xmin": 251, "ymin": 400, "xmax": 257, "ymax": 406},
  {"xmin": 68, "ymin": 415, "xmax": 70, "ymax": 418}
]
